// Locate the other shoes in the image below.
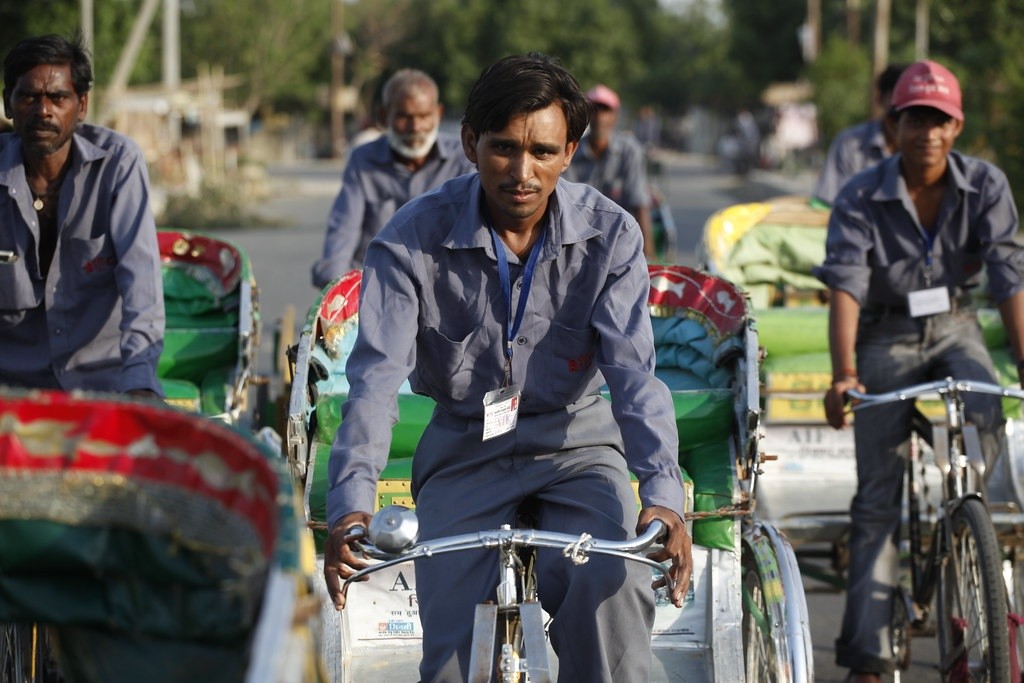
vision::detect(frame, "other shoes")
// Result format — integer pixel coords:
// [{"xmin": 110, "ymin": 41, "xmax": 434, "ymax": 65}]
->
[{"xmin": 846, "ymin": 670, "xmax": 880, "ymax": 683}]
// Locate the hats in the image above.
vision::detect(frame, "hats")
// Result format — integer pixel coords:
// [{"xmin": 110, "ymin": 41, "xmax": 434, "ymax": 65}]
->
[
  {"xmin": 889, "ymin": 61, "xmax": 964, "ymax": 121},
  {"xmin": 587, "ymin": 85, "xmax": 620, "ymax": 109}
]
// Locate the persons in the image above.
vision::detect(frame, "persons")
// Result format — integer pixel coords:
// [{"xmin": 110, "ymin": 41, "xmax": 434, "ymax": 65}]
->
[
  {"xmin": 313, "ymin": 70, "xmax": 478, "ymax": 288},
  {"xmin": 824, "ymin": 62, "xmax": 1023, "ymax": 683},
  {"xmin": 0, "ymin": 35, "xmax": 165, "ymax": 402},
  {"xmin": 324, "ymin": 53, "xmax": 693, "ymax": 683},
  {"xmin": 812, "ymin": 63, "xmax": 909, "ymax": 212},
  {"xmin": 563, "ymin": 85, "xmax": 653, "ymax": 257}
]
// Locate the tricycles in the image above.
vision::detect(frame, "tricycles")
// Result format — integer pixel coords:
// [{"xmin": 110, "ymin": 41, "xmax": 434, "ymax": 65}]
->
[
  {"xmin": 285, "ymin": 263, "xmax": 815, "ymax": 683},
  {"xmin": 699, "ymin": 195, "xmax": 1024, "ymax": 683},
  {"xmin": 2, "ymin": 381, "xmax": 323, "ymax": 683},
  {"xmin": 147, "ymin": 228, "xmax": 296, "ymax": 438}
]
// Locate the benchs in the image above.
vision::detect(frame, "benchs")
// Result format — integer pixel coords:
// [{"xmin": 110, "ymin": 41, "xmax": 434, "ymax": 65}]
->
[
  {"xmin": 755, "ymin": 308, "xmax": 1024, "ymax": 426},
  {"xmin": 305, "ymin": 390, "xmax": 738, "ymax": 552},
  {"xmin": 156, "ymin": 329, "xmax": 238, "ymax": 416}
]
